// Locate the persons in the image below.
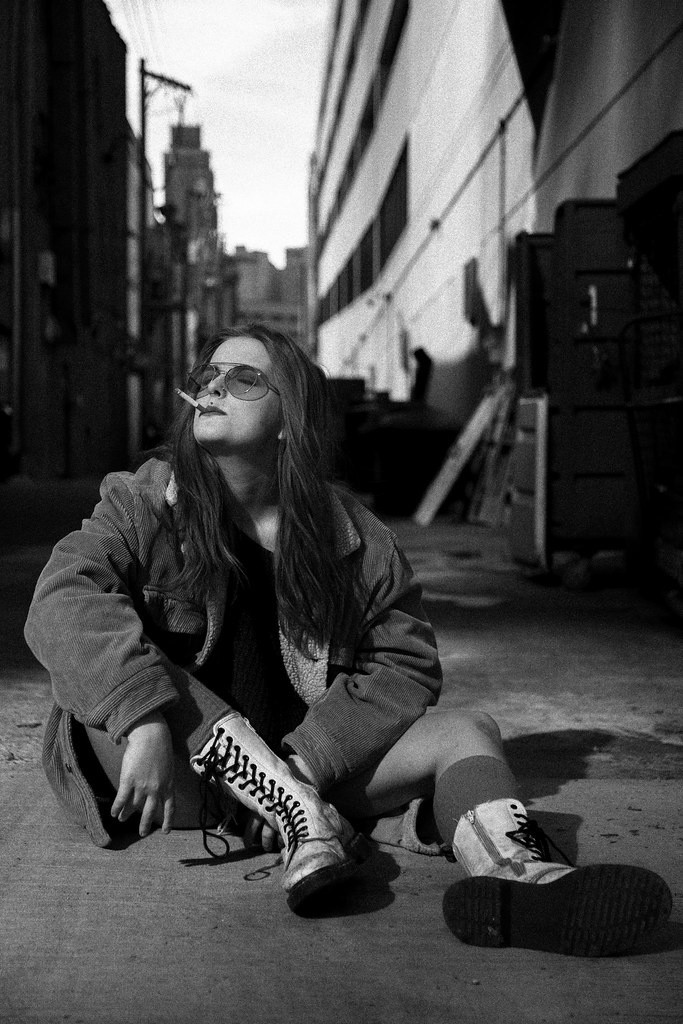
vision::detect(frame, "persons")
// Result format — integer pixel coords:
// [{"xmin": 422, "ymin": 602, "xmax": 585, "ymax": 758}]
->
[{"xmin": 20, "ymin": 323, "xmax": 672, "ymax": 959}]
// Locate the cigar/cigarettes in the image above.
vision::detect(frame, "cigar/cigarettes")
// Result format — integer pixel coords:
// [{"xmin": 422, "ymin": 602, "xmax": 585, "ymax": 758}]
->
[{"xmin": 172, "ymin": 385, "xmax": 206, "ymax": 415}]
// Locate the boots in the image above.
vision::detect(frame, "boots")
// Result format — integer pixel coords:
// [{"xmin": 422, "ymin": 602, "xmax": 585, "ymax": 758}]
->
[
  {"xmin": 442, "ymin": 798, "xmax": 673, "ymax": 957},
  {"xmin": 189, "ymin": 711, "xmax": 364, "ymax": 909}
]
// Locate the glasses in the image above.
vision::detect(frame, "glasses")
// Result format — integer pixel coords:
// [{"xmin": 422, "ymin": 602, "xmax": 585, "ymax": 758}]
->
[{"xmin": 187, "ymin": 362, "xmax": 282, "ymax": 395}]
